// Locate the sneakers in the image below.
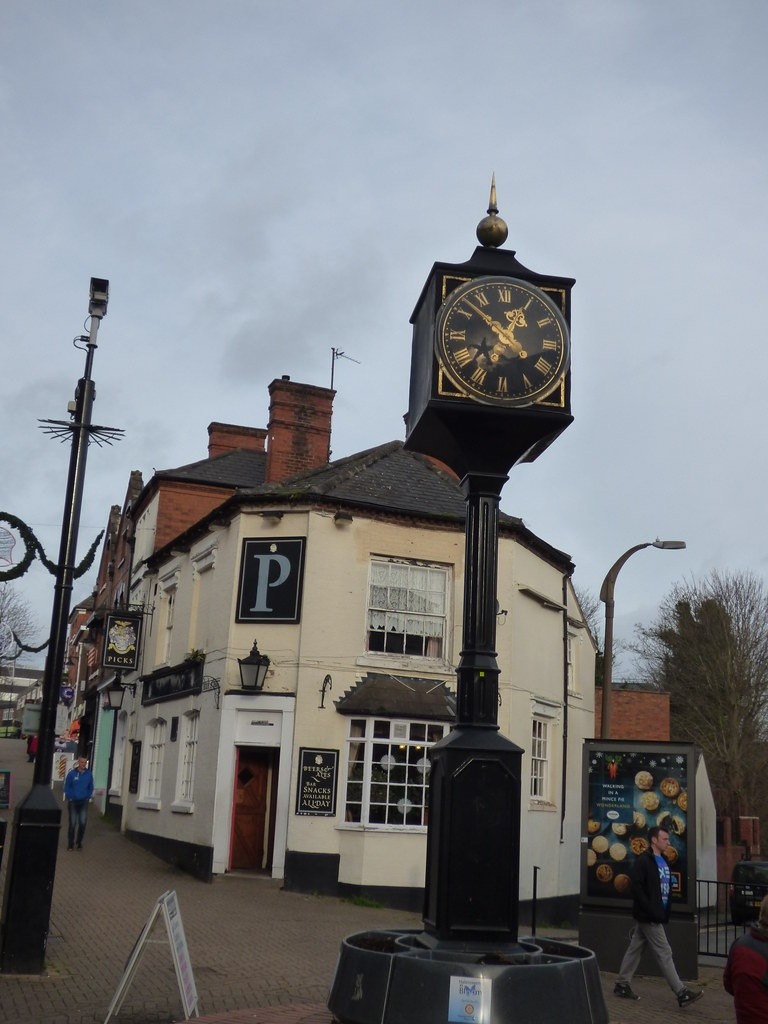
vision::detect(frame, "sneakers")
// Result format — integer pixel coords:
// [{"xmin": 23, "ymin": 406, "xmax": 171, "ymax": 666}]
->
[
  {"xmin": 613, "ymin": 982, "xmax": 641, "ymax": 1000},
  {"xmin": 677, "ymin": 989, "xmax": 704, "ymax": 1008}
]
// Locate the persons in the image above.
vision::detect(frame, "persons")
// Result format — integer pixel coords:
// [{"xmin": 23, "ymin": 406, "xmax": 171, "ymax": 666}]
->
[
  {"xmin": 26, "ymin": 734, "xmax": 38, "ymax": 763},
  {"xmin": 64, "ymin": 755, "xmax": 94, "ymax": 851},
  {"xmin": 723, "ymin": 894, "xmax": 768, "ymax": 1024},
  {"xmin": 613, "ymin": 825, "xmax": 705, "ymax": 1009}
]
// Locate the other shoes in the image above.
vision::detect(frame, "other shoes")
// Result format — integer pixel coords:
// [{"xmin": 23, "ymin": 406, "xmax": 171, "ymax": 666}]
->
[
  {"xmin": 76, "ymin": 841, "xmax": 83, "ymax": 849},
  {"xmin": 67, "ymin": 843, "xmax": 74, "ymax": 851}
]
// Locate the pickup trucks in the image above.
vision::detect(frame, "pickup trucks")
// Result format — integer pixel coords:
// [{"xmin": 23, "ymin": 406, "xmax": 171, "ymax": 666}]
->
[{"xmin": 728, "ymin": 860, "xmax": 768, "ymax": 926}]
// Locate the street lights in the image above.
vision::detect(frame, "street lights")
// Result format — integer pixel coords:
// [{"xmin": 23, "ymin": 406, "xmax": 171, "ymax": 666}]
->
[{"xmin": 598, "ymin": 538, "xmax": 687, "ymax": 737}]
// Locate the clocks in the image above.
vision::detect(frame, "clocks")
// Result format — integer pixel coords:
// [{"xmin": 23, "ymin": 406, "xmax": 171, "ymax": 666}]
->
[
  {"xmin": 107, "ymin": 670, "xmax": 137, "ymax": 709},
  {"xmin": 234, "ymin": 640, "xmax": 271, "ymax": 691},
  {"xmin": 431, "ymin": 275, "xmax": 570, "ymax": 407}
]
